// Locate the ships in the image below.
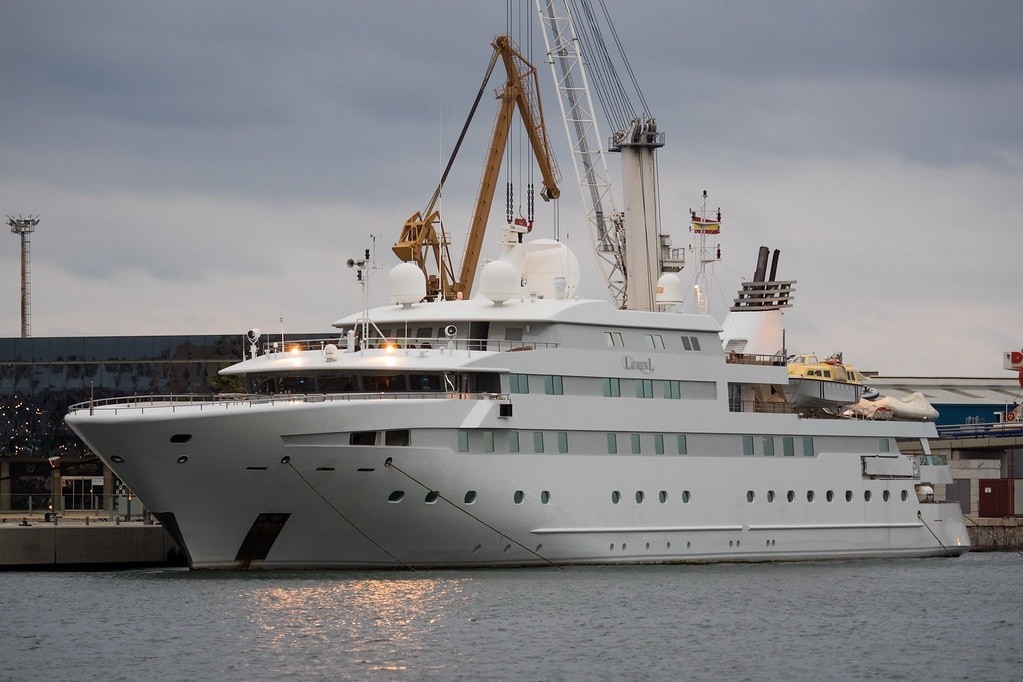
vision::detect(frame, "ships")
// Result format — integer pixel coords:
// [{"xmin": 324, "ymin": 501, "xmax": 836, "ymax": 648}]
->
[{"xmin": 64, "ymin": 0, "xmax": 972, "ymax": 571}]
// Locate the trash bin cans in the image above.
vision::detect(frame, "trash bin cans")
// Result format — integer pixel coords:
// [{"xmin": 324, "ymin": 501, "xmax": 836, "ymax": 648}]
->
[{"xmin": 119, "ymin": 495, "xmax": 143, "ymax": 517}]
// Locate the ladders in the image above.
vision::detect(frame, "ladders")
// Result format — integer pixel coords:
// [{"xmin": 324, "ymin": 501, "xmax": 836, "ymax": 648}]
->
[{"xmin": 536, "ymin": 0, "xmax": 628, "ymax": 309}]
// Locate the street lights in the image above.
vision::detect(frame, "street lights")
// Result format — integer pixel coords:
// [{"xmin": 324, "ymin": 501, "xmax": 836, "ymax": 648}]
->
[{"xmin": 48, "ymin": 457, "xmax": 60, "ymax": 512}]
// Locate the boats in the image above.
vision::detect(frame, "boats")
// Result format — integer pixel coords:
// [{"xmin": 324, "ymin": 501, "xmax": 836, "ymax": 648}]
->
[{"xmin": 772, "ymin": 351, "xmax": 868, "ymax": 408}]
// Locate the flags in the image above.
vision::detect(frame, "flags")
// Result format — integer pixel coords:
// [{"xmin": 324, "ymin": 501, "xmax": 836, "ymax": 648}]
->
[{"xmin": 694, "ymin": 217, "xmax": 719, "ymax": 234}]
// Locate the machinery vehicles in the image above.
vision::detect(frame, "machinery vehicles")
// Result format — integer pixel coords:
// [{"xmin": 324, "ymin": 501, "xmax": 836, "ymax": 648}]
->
[{"xmin": 391, "ymin": 34, "xmax": 563, "ymax": 302}]
